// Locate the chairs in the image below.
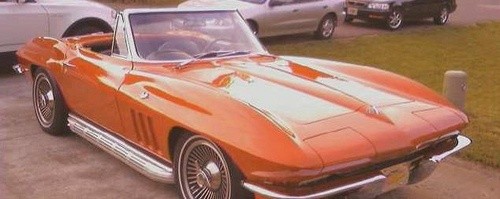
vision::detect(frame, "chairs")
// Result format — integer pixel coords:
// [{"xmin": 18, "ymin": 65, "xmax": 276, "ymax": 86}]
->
[{"xmin": 84, "ymin": 40, "xmax": 209, "ymax": 59}]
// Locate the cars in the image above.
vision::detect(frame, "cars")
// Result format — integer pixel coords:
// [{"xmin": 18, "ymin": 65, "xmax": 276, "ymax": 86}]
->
[
  {"xmin": 11, "ymin": 5, "xmax": 472, "ymax": 199},
  {"xmin": 345, "ymin": 1, "xmax": 457, "ymax": 30},
  {"xmin": 0, "ymin": 0, "xmax": 120, "ymax": 72},
  {"xmin": 176, "ymin": 1, "xmax": 344, "ymax": 40}
]
